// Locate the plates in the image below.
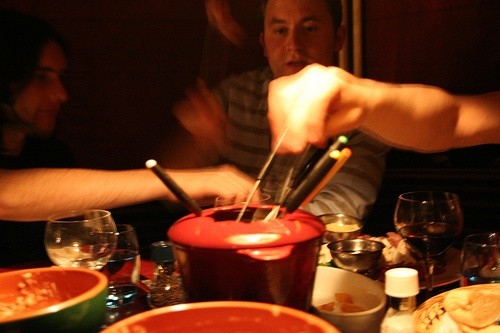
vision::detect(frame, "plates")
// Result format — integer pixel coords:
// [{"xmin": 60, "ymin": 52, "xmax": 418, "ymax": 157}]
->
[{"xmin": 409, "ymin": 283, "xmax": 500, "ymax": 333}]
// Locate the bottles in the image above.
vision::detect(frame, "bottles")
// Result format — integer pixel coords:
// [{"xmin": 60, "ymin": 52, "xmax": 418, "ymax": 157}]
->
[{"xmin": 381, "ymin": 268, "xmax": 420, "ymax": 333}]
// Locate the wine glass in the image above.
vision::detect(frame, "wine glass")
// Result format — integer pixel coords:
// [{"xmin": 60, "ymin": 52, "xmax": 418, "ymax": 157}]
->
[{"xmin": 394, "ymin": 190, "xmax": 463, "ymax": 301}]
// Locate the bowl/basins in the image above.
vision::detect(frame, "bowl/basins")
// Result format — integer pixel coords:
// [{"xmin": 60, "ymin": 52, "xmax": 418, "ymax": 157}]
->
[
  {"xmin": 310, "ymin": 266, "xmax": 387, "ymax": 333},
  {"xmin": 327, "ymin": 239, "xmax": 386, "ymax": 271},
  {"xmin": 0, "ymin": 267, "xmax": 108, "ymax": 333},
  {"xmin": 101, "ymin": 301, "xmax": 339, "ymax": 333},
  {"xmin": 317, "ymin": 213, "xmax": 365, "ymax": 243}
]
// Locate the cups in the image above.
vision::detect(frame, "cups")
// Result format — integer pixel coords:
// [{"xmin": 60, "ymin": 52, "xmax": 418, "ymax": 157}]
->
[
  {"xmin": 146, "ymin": 239, "xmax": 174, "ymax": 276},
  {"xmin": 213, "ymin": 193, "xmax": 271, "ymax": 206},
  {"xmin": 460, "ymin": 232, "xmax": 500, "ymax": 286},
  {"xmin": 91, "ymin": 223, "xmax": 140, "ymax": 289},
  {"xmin": 44, "ymin": 209, "xmax": 118, "ymax": 272},
  {"xmin": 166, "ymin": 204, "xmax": 325, "ymax": 312},
  {"xmin": 104, "ymin": 281, "xmax": 152, "ymax": 323}
]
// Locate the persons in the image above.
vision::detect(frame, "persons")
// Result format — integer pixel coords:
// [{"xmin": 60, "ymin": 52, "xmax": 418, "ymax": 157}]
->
[
  {"xmin": 0, "ymin": 22, "xmax": 260, "ymax": 271},
  {"xmin": 159, "ymin": 0, "xmax": 389, "ymax": 220},
  {"xmin": 267, "ymin": 63, "xmax": 500, "ymax": 155}
]
